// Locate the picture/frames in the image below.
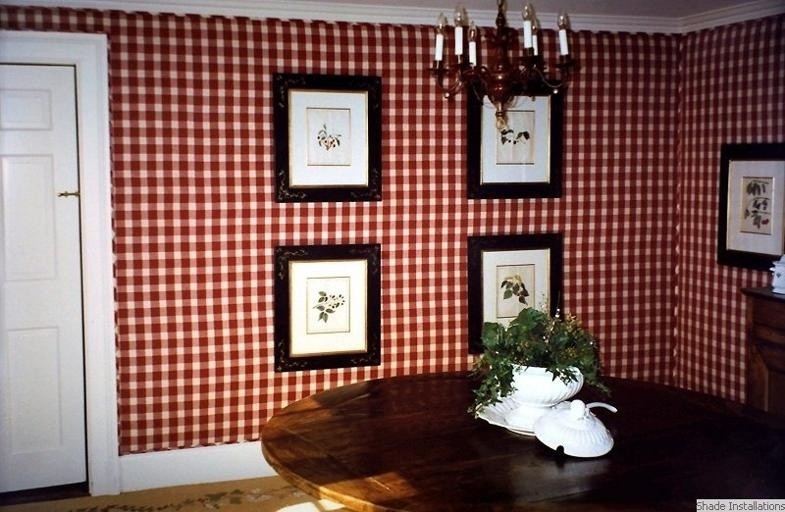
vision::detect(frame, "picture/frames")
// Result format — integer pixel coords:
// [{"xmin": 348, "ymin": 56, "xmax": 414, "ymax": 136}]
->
[
  {"xmin": 270, "ymin": 71, "xmax": 384, "ymax": 204},
  {"xmin": 465, "ymin": 70, "xmax": 564, "ymax": 200},
  {"xmin": 273, "ymin": 243, "xmax": 383, "ymax": 373},
  {"xmin": 465, "ymin": 231, "xmax": 565, "ymax": 357},
  {"xmin": 717, "ymin": 141, "xmax": 785, "ymax": 272}
]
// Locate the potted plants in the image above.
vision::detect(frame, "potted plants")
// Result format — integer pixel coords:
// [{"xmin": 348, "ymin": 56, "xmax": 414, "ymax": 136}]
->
[{"xmin": 460, "ymin": 305, "xmax": 614, "ymax": 434}]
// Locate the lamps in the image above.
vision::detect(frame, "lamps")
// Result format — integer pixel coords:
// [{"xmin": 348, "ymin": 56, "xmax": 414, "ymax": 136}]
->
[{"xmin": 426, "ymin": 0, "xmax": 577, "ymax": 131}]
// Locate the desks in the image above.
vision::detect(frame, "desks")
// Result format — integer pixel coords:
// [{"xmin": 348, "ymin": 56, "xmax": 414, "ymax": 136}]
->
[{"xmin": 259, "ymin": 369, "xmax": 785, "ymax": 512}]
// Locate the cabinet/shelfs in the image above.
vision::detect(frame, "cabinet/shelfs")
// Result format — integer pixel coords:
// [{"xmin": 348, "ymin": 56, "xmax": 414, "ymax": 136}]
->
[{"xmin": 739, "ymin": 286, "xmax": 785, "ymax": 420}]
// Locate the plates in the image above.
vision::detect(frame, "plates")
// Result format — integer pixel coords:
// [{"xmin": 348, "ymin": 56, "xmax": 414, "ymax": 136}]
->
[{"xmin": 479, "ymin": 394, "xmax": 596, "ymax": 437}]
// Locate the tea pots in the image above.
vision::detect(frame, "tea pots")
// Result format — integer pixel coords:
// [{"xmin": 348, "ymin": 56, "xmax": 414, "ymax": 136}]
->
[{"xmin": 770, "ymin": 252, "xmax": 785, "ymax": 294}]
